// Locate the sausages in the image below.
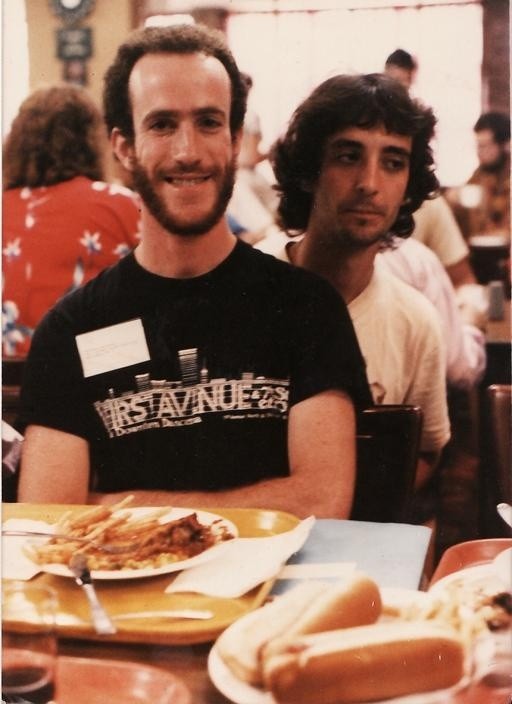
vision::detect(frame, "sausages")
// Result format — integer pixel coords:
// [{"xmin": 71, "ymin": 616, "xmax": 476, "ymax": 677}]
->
[
  {"xmin": 250, "ymin": 577, "xmax": 468, "ymax": 701},
  {"xmin": 71, "ymin": 504, "xmax": 113, "ymax": 530}
]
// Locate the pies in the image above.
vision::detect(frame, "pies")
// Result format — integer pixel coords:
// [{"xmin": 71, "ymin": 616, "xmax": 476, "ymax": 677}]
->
[{"xmin": 36, "ymin": 512, "xmax": 201, "ymax": 573}]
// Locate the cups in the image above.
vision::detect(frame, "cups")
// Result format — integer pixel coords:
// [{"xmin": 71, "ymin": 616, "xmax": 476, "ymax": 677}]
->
[{"xmin": 2, "ymin": 585, "xmax": 57, "ymax": 704}]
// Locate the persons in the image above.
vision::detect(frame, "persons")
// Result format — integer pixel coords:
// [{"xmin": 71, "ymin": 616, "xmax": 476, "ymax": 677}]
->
[
  {"xmin": 222, "ymin": 48, "xmax": 511, "ymax": 494},
  {"xmin": 15, "ymin": 23, "xmax": 375, "ymax": 522},
  {"xmin": 2, "ymin": 85, "xmax": 144, "ymax": 360}
]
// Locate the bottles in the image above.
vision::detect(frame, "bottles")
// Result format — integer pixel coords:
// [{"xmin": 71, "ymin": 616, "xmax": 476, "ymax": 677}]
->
[{"xmin": 486, "ymin": 281, "xmax": 506, "ymax": 321}]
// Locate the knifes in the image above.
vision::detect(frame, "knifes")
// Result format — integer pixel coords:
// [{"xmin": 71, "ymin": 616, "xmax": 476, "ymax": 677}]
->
[{"xmin": 64, "ymin": 551, "xmax": 119, "ymax": 637}]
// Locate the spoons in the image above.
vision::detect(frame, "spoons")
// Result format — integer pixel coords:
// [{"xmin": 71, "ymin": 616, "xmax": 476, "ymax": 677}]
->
[{"xmin": 2, "ymin": 531, "xmax": 140, "ymax": 554}]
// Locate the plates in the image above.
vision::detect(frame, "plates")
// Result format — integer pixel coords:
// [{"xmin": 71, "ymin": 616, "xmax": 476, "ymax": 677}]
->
[
  {"xmin": 207, "ymin": 583, "xmax": 495, "ymax": 704},
  {"xmin": 31, "ymin": 507, "xmax": 240, "ymax": 578}
]
